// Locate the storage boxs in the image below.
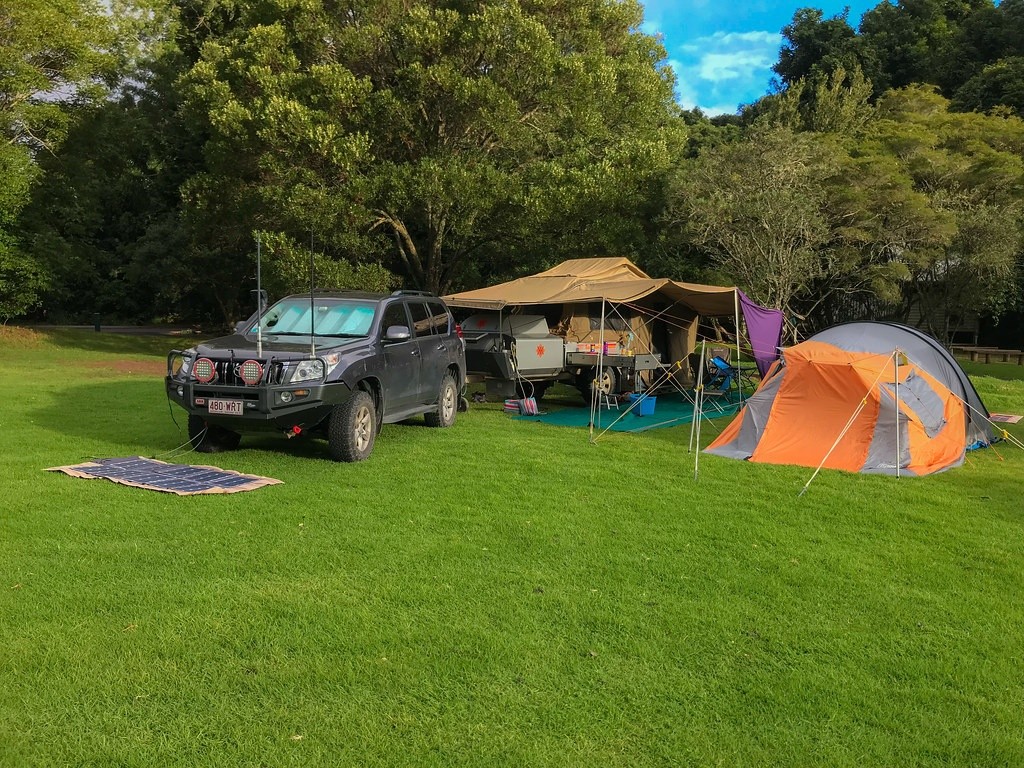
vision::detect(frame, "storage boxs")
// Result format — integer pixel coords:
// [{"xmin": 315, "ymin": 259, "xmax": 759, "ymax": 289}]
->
[{"xmin": 630, "ymin": 393, "xmax": 657, "ymax": 417}]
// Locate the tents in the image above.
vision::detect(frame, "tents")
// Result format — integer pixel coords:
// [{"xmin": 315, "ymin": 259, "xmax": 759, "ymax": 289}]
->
[
  {"xmin": 437, "ymin": 256, "xmax": 785, "ymax": 454},
  {"xmin": 698, "ymin": 320, "xmax": 1023, "ymax": 503}
]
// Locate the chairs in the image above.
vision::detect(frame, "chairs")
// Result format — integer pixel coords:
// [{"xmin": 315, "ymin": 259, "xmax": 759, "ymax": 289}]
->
[
  {"xmin": 681, "ymin": 352, "xmax": 730, "ymax": 403},
  {"xmin": 708, "ymin": 356, "xmax": 759, "ymax": 403}
]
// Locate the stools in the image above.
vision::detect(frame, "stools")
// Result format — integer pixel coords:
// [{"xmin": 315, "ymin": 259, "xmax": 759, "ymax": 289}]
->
[
  {"xmin": 598, "ymin": 393, "xmax": 619, "ymax": 410},
  {"xmin": 700, "ymin": 390, "xmax": 725, "ymax": 413}
]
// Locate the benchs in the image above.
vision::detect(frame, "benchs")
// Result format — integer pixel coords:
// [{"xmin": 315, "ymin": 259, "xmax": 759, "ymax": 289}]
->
[{"xmin": 946, "ymin": 343, "xmax": 1024, "ymax": 366}]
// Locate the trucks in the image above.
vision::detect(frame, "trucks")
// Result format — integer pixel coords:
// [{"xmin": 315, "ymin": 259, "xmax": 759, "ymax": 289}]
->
[{"xmin": 460, "ymin": 292, "xmax": 696, "ymax": 407}]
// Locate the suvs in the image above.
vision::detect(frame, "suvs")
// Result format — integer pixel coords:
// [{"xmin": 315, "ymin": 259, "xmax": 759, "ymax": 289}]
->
[{"xmin": 164, "ymin": 289, "xmax": 467, "ymax": 462}]
